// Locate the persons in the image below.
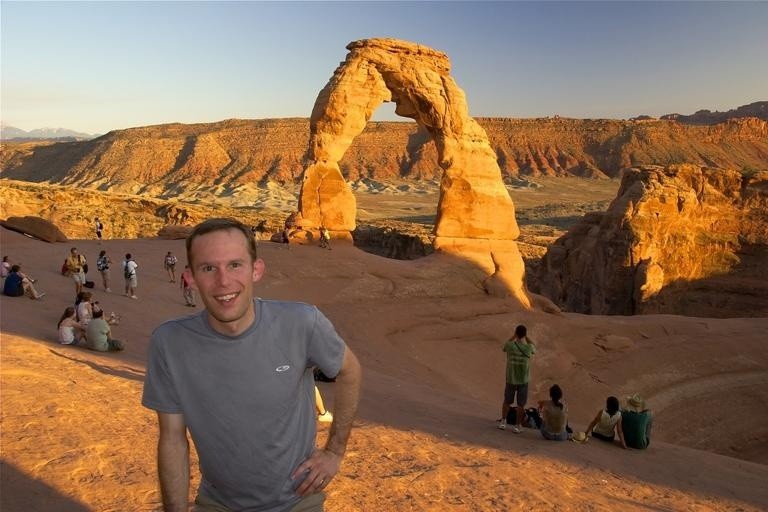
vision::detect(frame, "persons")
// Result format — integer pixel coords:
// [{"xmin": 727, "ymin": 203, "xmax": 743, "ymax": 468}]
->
[
  {"xmin": 122, "ymin": 253, "xmax": 140, "ymax": 299},
  {"xmin": 280, "ymin": 227, "xmax": 290, "ymax": 250},
  {"xmin": 539, "ymin": 384, "xmax": 569, "ymax": 441},
  {"xmin": 55, "ymin": 247, "xmax": 127, "ymax": 353},
  {"xmin": 95, "ymin": 217, "xmax": 103, "ymax": 240},
  {"xmin": 622, "ymin": 392, "xmax": 652, "ymax": 449},
  {"xmin": 586, "ymin": 396, "xmax": 632, "ymax": 452},
  {"xmin": 250, "ymin": 224, "xmax": 259, "ymax": 248},
  {"xmin": 314, "ymin": 384, "xmax": 334, "ymax": 423},
  {"xmin": 1, "ymin": 255, "xmax": 46, "ymax": 302},
  {"xmin": 180, "ymin": 265, "xmax": 198, "ymax": 307},
  {"xmin": 323, "ymin": 226, "xmax": 332, "ymax": 250},
  {"xmin": 164, "ymin": 252, "xmax": 178, "ymax": 283},
  {"xmin": 499, "ymin": 325, "xmax": 536, "ymax": 433},
  {"xmin": 318, "ymin": 227, "xmax": 324, "ymax": 247},
  {"xmin": 143, "ymin": 219, "xmax": 362, "ymax": 512}
]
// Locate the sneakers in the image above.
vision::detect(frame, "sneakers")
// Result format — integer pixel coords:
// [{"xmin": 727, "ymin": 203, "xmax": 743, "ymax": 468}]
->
[
  {"xmin": 496, "ymin": 424, "xmax": 504, "ymax": 430},
  {"xmin": 512, "ymin": 424, "xmax": 524, "ymax": 432}
]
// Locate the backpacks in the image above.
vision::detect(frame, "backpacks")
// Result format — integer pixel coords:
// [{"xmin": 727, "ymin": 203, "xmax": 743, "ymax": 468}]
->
[{"xmin": 507, "ymin": 406, "xmax": 542, "ymax": 429}]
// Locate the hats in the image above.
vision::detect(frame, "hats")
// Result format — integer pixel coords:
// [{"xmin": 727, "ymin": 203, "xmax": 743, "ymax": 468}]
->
[
  {"xmin": 91, "ymin": 301, "xmax": 102, "ymax": 318},
  {"xmin": 572, "ymin": 432, "xmax": 589, "ymax": 442},
  {"xmin": 626, "ymin": 393, "xmax": 646, "ymax": 411}
]
